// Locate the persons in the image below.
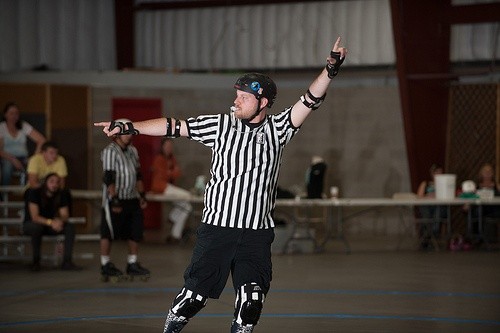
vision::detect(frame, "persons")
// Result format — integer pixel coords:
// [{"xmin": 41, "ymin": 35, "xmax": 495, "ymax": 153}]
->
[
  {"xmin": 93, "ymin": 36, "xmax": 349, "ymax": 333},
  {"xmin": 0, "ymin": 101, "xmax": 500, "ymax": 283}
]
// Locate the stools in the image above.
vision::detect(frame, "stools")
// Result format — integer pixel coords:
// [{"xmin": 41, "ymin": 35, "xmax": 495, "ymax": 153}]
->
[
  {"xmin": 473, "ymin": 217, "xmax": 500, "ymax": 250},
  {"xmin": 415, "ymin": 217, "xmax": 448, "ymax": 251}
]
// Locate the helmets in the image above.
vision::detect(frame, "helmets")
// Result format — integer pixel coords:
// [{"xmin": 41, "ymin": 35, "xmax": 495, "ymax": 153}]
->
[{"xmin": 234, "ymin": 73, "xmax": 277, "ymax": 108}]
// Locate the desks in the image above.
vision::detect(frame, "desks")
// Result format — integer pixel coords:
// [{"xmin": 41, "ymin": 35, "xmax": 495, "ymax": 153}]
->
[{"xmin": 147, "ymin": 195, "xmax": 500, "ymax": 256}]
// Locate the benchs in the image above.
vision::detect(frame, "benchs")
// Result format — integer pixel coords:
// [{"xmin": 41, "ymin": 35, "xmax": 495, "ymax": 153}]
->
[{"xmin": 0, "ymin": 185, "xmax": 101, "ymax": 261}]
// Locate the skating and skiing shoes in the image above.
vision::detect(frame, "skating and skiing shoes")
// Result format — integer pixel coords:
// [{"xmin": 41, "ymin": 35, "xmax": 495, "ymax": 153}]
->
[
  {"xmin": 126, "ymin": 262, "xmax": 150, "ymax": 281},
  {"xmin": 99, "ymin": 262, "xmax": 123, "ymax": 283}
]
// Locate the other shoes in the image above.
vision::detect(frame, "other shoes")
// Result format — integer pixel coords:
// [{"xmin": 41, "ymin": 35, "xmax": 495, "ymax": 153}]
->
[
  {"xmin": 28, "ymin": 262, "xmax": 39, "ymax": 271},
  {"xmin": 63, "ymin": 262, "xmax": 80, "ymax": 269}
]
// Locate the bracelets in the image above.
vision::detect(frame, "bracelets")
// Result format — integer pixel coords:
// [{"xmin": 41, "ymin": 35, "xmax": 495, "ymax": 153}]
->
[{"xmin": 47, "ymin": 219, "xmax": 51, "ymax": 227}]
